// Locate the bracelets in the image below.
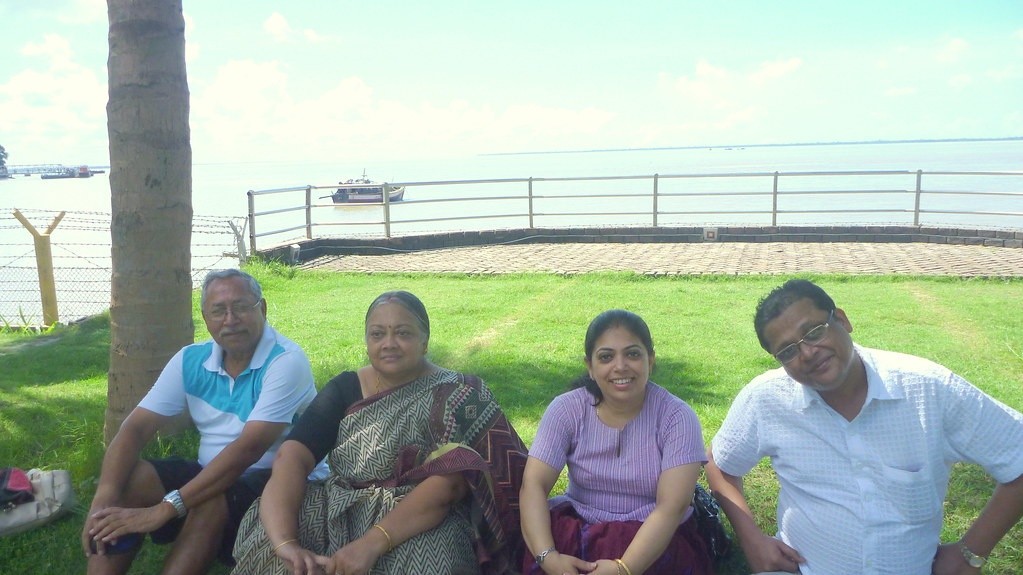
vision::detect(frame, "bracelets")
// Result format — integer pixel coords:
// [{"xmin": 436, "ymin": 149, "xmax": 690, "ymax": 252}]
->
[
  {"xmin": 534, "ymin": 546, "xmax": 557, "ymax": 566},
  {"xmin": 372, "ymin": 524, "xmax": 393, "ymax": 553},
  {"xmin": 273, "ymin": 539, "xmax": 300, "ymax": 551},
  {"xmin": 614, "ymin": 558, "xmax": 632, "ymax": 575}
]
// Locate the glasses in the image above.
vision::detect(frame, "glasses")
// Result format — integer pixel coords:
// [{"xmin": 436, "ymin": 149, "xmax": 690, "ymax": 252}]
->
[
  {"xmin": 776, "ymin": 308, "xmax": 835, "ymax": 365},
  {"xmin": 202, "ymin": 299, "xmax": 260, "ymax": 323}
]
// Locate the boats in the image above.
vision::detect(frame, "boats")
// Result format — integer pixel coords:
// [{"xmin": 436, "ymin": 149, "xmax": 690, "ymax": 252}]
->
[
  {"xmin": 317, "ymin": 167, "xmax": 404, "ymax": 204},
  {"xmin": 40, "ymin": 164, "xmax": 95, "ymax": 178}
]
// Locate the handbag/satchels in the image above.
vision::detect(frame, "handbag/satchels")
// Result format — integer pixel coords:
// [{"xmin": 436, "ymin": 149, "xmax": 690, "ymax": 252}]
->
[{"xmin": 695, "ymin": 482, "xmax": 734, "ymax": 561}]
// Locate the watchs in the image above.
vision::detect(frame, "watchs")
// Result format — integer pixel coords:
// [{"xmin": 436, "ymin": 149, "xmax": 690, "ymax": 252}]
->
[
  {"xmin": 160, "ymin": 489, "xmax": 188, "ymax": 518},
  {"xmin": 958, "ymin": 539, "xmax": 987, "ymax": 569}
]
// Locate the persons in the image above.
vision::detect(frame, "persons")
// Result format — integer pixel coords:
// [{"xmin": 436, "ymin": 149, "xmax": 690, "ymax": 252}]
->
[
  {"xmin": 519, "ymin": 308, "xmax": 717, "ymax": 575},
  {"xmin": 82, "ymin": 265, "xmax": 320, "ymax": 575},
  {"xmin": 227, "ymin": 289, "xmax": 519, "ymax": 575},
  {"xmin": 705, "ymin": 278, "xmax": 1023, "ymax": 575}
]
